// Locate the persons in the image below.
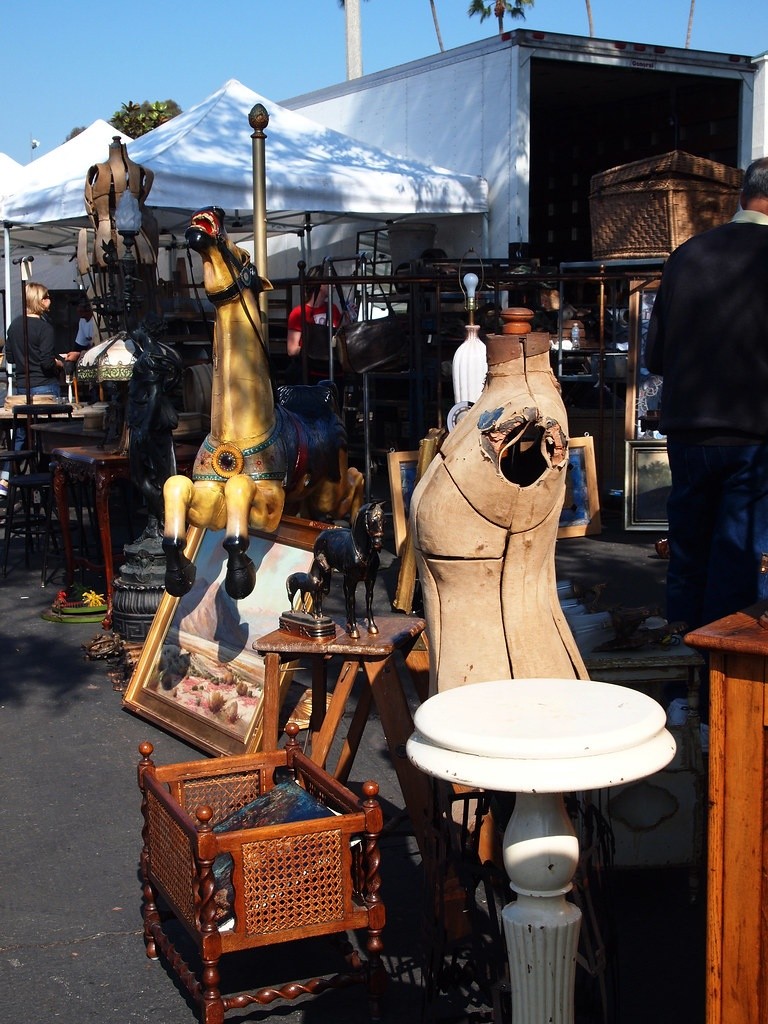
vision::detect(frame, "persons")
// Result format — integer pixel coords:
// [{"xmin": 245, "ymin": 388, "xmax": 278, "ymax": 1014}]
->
[
  {"xmin": 0, "ymin": 283, "xmax": 61, "ymax": 497},
  {"xmin": 64, "ymin": 296, "xmax": 113, "ymax": 361},
  {"xmin": 287, "ymin": 265, "xmax": 342, "ymax": 357},
  {"xmin": 643, "ymin": 158, "xmax": 768, "ymax": 726}
]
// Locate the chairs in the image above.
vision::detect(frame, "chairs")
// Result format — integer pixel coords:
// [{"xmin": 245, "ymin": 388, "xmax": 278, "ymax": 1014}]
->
[{"xmin": 0, "ymin": 400, "xmax": 109, "ymax": 576}]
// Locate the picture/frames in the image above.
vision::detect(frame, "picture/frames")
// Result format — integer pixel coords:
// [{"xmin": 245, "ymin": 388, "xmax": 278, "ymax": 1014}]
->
[
  {"xmin": 518, "ymin": 433, "xmax": 602, "ymax": 538},
  {"xmin": 624, "ymin": 279, "xmax": 668, "ymax": 442},
  {"xmin": 623, "ymin": 439, "xmax": 673, "ymax": 532},
  {"xmin": 387, "ymin": 447, "xmax": 424, "ymax": 556},
  {"xmin": 120, "ymin": 508, "xmax": 347, "ymax": 757}
]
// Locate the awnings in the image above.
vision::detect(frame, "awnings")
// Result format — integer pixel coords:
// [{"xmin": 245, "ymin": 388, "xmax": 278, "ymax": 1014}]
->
[{"xmin": 0, "ymin": 78, "xmax": 490, "ymax": 224}]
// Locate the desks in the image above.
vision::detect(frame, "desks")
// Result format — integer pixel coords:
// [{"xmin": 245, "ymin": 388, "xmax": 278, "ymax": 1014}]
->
[
  {"xmin": 29, "ymin": 417, "xmax": 111, "ymax": 474},
  {"xmin": 48, "ymin": 439, "xmax": 199, "ymax": 630},
  {"xmin": 255, "ymin": 612, "xmax": 439, "ymax": 880}
]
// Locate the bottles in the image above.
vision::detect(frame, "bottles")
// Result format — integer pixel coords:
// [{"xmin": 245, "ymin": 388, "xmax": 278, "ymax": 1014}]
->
[{"xmin": 572, "ymin": 323, "xmax": 580, "ymax": 350}]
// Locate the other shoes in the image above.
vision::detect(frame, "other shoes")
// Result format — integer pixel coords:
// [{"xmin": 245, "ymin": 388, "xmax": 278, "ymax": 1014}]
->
[{"xmin": 0, "ymin": 480, "xmax": 8, "ymax": 496}]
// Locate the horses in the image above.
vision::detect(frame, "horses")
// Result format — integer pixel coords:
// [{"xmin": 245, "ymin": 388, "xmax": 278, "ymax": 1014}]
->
[
  {"xmin": 160, "ymin": 206, "xmax": 366, "ymax": 599},
  {"xmin": 287, "ymin": 499, "xmax": 387, "ymax": 641}
]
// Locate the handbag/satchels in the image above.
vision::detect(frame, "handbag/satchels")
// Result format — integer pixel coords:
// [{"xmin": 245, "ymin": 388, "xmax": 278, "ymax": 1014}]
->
[
  {"xmin": 336, "ymin": 313, "xmax": 413, "ymax": 376},
  {"xmin": 306, "ymin": 322, "xmax": 338, "ymax": 378}
]
